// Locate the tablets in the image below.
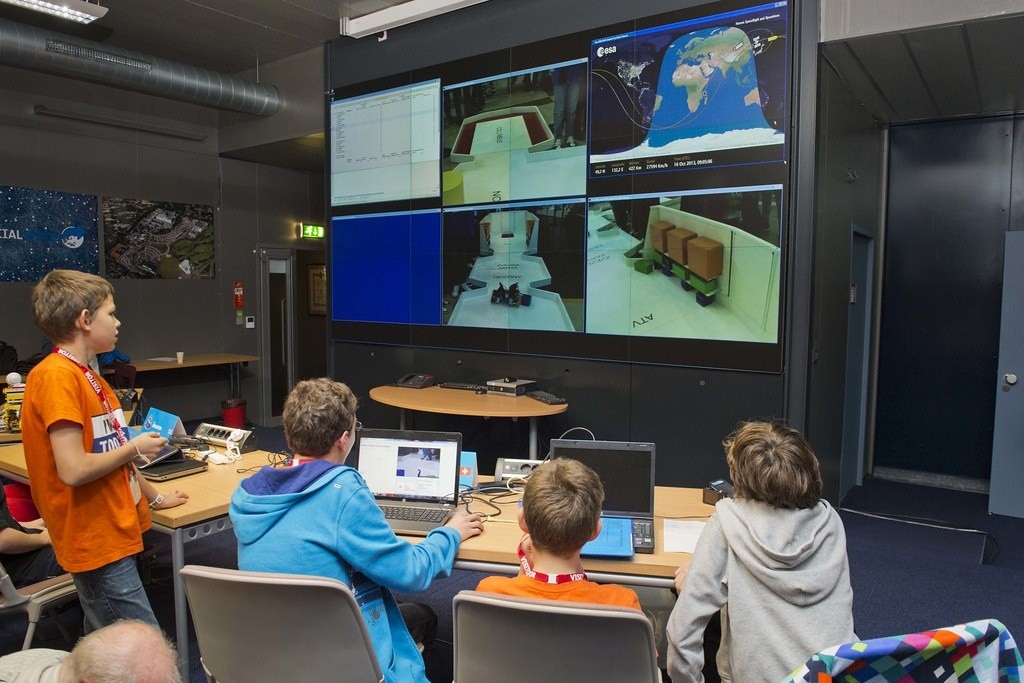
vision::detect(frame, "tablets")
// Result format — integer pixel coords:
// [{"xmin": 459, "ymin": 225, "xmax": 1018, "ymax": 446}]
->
[{"xmin": 132, "ymin": 446, "xmax": 179, "ymax": 468}]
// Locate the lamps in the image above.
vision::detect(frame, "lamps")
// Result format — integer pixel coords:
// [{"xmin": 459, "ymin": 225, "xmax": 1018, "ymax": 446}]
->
[{"xmin": 0, "ymin": 0, "xmax": 107, "ymax": 25}]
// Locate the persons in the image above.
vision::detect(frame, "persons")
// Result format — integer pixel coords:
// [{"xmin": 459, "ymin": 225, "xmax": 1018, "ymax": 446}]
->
[
  {"xmin": 0, "ymin": 481, "xmax": 85, "ymax": 657},
  {"xmin": 228, "ymin": 378, "xmax": 484, "ymax": 683},
  {"xmin": 665, "ymin": 423, "xmax": 862, "ymax": 683},
  {"xmin": 21, "ymin": 269, "xmax": 189, "ymax": 638},
  {"xmin": 476, "ymin": 458, "xmax": 658, "ymax": 657},
  {"xmin": 0, "ymin": 620, "xmax": 181, "ymax": 683}
]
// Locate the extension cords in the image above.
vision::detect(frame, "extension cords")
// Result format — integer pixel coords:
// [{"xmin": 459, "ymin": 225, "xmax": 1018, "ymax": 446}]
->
[
  {"xmin": 194, "ymin": 422, "xmax": 246, "ymax": 449},
  {"xmin": 197, "ymin": 450, "xmax": 229, "ymax": 464},
  {"xmin": 501, "ymin": 459, "xmax": 551, "ymax": 482}
]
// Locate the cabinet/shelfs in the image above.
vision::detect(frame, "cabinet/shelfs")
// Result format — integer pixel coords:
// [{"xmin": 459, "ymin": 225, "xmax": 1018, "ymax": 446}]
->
[{"xmin": 650, "ymin": 243, "xmax": 722, "ymax": 298}]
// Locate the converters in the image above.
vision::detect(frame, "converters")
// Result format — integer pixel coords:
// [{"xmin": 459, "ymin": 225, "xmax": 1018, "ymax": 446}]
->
[{"xmin": 477, "ymin": 480, "xmax": 513, "ymax": 494}]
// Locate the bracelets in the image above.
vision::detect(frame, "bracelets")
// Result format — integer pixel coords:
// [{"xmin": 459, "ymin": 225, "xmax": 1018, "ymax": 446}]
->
[{"xmin": 149, "ymin": 495, "xmax": 164, "ymax": 508}]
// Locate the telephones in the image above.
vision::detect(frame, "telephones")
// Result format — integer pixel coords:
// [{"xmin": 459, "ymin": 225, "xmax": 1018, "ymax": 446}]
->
[{"xmin": 397, "ymin": 373, "xmax": 436, "ymax": 388}]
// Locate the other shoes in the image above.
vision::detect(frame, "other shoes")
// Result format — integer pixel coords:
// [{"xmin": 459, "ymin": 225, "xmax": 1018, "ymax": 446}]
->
[
  {"xmin": 555, "ymin": 140, "xmax": 562, "ymax": 150},
  {"xmin": 565, "ymin": 136, "xmax": 577, "ymax": 146}
]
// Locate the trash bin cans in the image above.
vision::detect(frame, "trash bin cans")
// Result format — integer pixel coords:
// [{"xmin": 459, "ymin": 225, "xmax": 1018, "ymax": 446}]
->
[{"xmin": 222, "ymin": 399, "xmax": 246, "ymax": 429}]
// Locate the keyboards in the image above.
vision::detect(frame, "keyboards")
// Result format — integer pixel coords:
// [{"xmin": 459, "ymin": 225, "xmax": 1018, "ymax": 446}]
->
[
  {"xmin": 438, "ymin": 383, "xmax": 487, "ymax": 391},
  {"xmin": 526, "ymin": 390, "xmax": 567, "ymax": 405}
]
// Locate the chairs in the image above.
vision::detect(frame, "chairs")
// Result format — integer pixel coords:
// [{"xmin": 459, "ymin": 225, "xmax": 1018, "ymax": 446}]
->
[
  {"xmin": 779, "ymin": 616, "xmax": 1024, "ymax": 683},
  {"xmin": 0, "ymin": 425, "xmax": 293, "ymax": 683},
  {"xmin": 452, "ymin": 590, "xmax": 662, "ymax": 683},
  {"xmin": 180, "ymin": 562, "xmax": 384, "ymax": 683},
  {"xmin": 0, "ymin": 560, "xmax": 80, "ymax": 652}
]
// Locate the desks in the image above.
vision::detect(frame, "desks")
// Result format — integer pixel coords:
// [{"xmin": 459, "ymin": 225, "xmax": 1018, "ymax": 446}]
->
[
  {"xmin": 124, "ymin": 350, "xmax": 267, "ymax": 404},
  {"xmin": 384, "ymin": 473, "xmax": 729, "ymax": 586},
  {"xmin": 367, "ymin": 381, "xmax": 572, "ymax": 461}
]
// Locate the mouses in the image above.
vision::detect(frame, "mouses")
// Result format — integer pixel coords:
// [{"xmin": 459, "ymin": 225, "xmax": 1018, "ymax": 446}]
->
[{"xmin": 475, "ymin": 389, "xmax": 488, "ymax": 393}]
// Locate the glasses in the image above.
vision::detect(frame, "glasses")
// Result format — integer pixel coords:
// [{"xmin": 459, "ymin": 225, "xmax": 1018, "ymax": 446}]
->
[{"xmin": 348, "ymin": 422, "xmax": 363, "ymax": 433}]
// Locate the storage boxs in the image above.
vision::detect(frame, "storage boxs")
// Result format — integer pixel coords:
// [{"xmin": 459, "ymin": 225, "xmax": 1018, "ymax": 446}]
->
[
  {"xmin": 695, "ymin": 291, "xmax": 714, "ymax": 308},
  {"xmin": 686, "ymin": 236, "xmax": 724, "ymax": 279},
  {"xmin": 633, "ymin": 260, "xmax": 653, "ymax": 275},
  {"xmin": 665, "ymin": 227, "xmax": 697, "ymax": 265},
  {"xmin": 649, "ymin": 220, "xmax": 676, "ymax": 254},
  {"xmin": 680, "ymin": 280, "xmax": 693, "ymax": 291},
  {"xmin": 672, "ymin": 263, "xmax": 689, "ymax": 280},
  {"xmin": 663, "ymin": 267, "xmax": 671, "ymax": 276},
  {"xmin": 646, "ymin": 246, "xmax": 669, "ymax": 265},
  {"xmin": 689, "ymin": 274, "xmax": 716, "ymax": 293}
]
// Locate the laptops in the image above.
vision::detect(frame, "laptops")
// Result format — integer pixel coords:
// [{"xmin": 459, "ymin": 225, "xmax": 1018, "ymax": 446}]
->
[
  {"xmin": 355, "ymin": 428, "xmax": 462, "ymax": 536},
  {"xmin": 135, "ymin": 457, "xmax": 209, "ymax": 480},
  {"xmin": 548, "ymin": 438, "xmax": 656, "ymax": 554}
]
